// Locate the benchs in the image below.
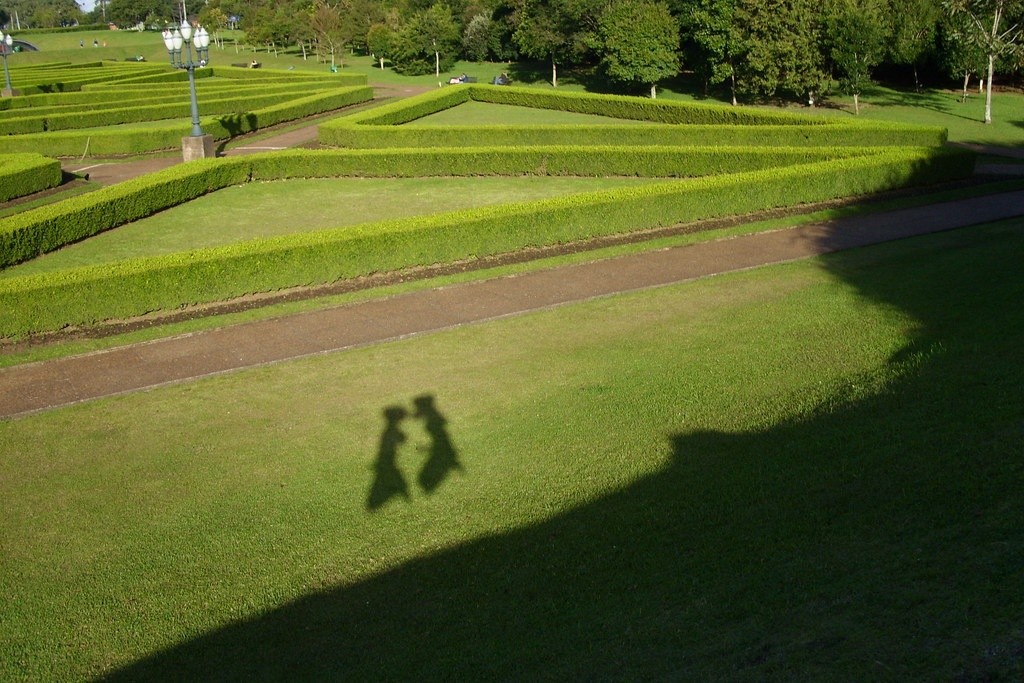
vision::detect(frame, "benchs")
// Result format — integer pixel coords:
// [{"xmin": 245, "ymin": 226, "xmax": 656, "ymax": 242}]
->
[
  {"xmin": 445, "ymin": 77, "xmax": 478, "ymax": 85},
  {"xmin": 231, "ymin": 63, "xmax": 247, "ymax": 67},
  {"xmin": 489, "ymin": 77, "xmax": 513, "ymax": 85},
  {"xmin": 125, "ymin": 58, "xmax": 145, "ymax": 62}
]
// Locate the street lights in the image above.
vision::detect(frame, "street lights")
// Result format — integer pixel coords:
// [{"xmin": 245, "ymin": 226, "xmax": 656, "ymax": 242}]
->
[
  {"xmin": 161, "ymin": 19, "xmax": 211, "ymax": 137},
  {"xmin": 0, "ymin": 31, "xmax": 13, "ymax": 90}
]
